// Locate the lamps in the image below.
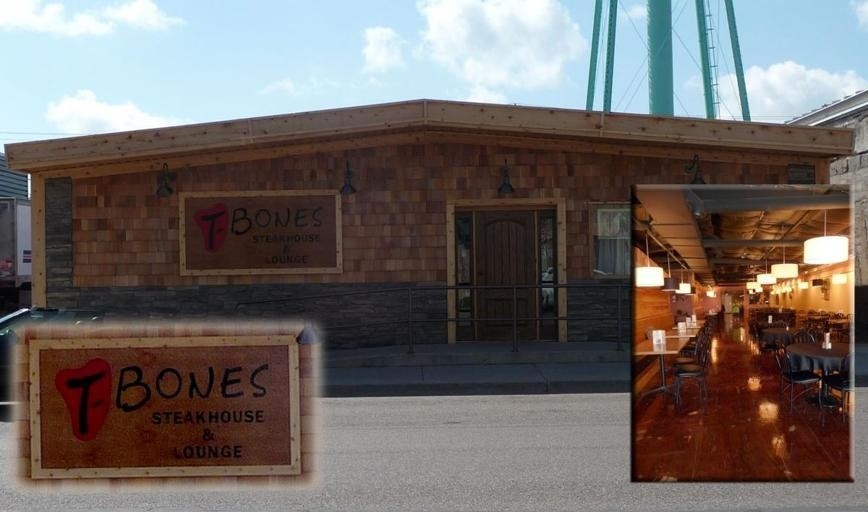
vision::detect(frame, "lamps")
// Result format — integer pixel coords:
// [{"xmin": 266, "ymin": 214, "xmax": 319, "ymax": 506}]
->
[
  {"xmin": 157, "ymin": 162, "xmax": 177, "ymax": 198},
  {"xmin": 688, "ymin": 155, "xmax": 705, "ymax": 187},
  {"xmin": 746, "ymin": 255, "xmax": 776, "ymax": 295},
  {"xmin": 340, "ymin": 160, "xmax": 357, "ymax": 195},
  {"xmin": 635, "ymin": 229, "xmax": 698, "ymax": 294},
  {"xmin": 498, "ymin": 158, "xmax": 515, "ymax": 195},
  {"xmin": 771, "ymin": 236, "xmax": 798, "ymax": 279},
  {"xmin": 802, "ymin": 211, "xmax": 849, "ymax": 265}
]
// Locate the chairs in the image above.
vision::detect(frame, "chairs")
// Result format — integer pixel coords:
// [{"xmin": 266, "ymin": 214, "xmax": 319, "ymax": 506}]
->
[
  {"xmin": 633, "ymin": 307, "xmax": 720, "ymax": 407},
  {"xmin": 747, "ymin": 305, "xmax": 849, "ymax": 424}
]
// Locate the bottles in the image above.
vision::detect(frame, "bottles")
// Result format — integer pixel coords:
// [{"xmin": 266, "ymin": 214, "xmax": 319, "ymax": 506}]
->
[{"xmin": 821, "ymin": 332, "xmax": 834, "ymax": 350}]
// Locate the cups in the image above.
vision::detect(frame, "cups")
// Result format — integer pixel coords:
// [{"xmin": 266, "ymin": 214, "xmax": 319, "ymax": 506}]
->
[
  {"xmin": 678, "ymin": 322, "xmax": 687, "ymax": 332},
  {"xmin": 652, "ymin": 329, "xmax": 666, "ymax": 345},
  {"xmin": 767, "ymin": 316, "xmax": 772, "ymax": 324},
  {"xmin": 685, "ymin": 314, "xmax": 696, "ymax": 322}
]
